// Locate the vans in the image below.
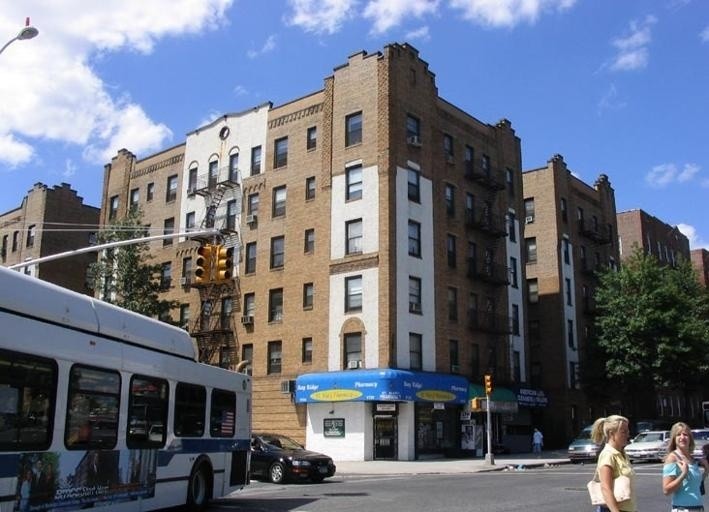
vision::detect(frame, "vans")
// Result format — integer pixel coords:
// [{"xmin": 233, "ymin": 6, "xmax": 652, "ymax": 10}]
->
[{"xmin": 567, "ymin": 424, "xmax": 605, "ymax": 464}]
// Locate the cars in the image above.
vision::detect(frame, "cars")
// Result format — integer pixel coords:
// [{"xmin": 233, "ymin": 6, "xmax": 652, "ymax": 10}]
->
[
  {"xmin": 248, "ymin": 431, "xmax": 337, "ymax": 484},
  {"xmin": 690, "ymin": 428, "xmax": 708, "ymax": 459},
  {"xmin": 623, "ymin": 430, "xmax": 672, "ymax": 464}
]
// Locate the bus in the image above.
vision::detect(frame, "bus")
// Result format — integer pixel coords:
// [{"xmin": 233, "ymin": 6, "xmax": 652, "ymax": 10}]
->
[{"xmin": 1, "ymin": 267, "xmax": 253, "ymax": 511}]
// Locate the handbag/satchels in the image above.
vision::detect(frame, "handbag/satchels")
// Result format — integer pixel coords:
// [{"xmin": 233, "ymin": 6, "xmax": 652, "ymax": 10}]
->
[{"xmin": 585, "ymin": 455, "xmax": 632, "ymax": 506}]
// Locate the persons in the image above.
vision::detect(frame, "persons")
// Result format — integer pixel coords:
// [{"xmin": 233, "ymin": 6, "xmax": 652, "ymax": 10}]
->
[
  {"xmin": 531, "ymin": 426, "xmax": 544, "ymax": 459},
  {"xmin": 19, "ymin": 469, "xmax": 34, "ymax": 511},
  {"xmin": 661, "ymin": 421, "xmax": 709, "ymax": 512},
  {"xmin": 589, "ymin": 414, "xmax": 639, "ymax": 512},
  {"xmin": 249, "ymin": 439, "xmax": 264, "ymax": 452},
  {"xmin": 80, "ymin": 449, "xmax": 105, "ymax": 508},
  {"xmin": 41, "ymin": 463, "xmax": 55, "ymax": 512},
  {"xmin": 30, "ymin": 459, "xmax": 46, "ymax": 511}
]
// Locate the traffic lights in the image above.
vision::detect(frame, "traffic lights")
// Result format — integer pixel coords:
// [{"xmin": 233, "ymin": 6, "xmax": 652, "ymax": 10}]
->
[
  {"xmin": 194, "ymin": 243, "xmax": 214, "ymax": 286},
  {"xmin": 484, "ymin": 375, "xmax": 494, "ymax": 394},
  {"xmin": 215, "ymin": 245, "xmax": 235, "ymax": 285}
]
